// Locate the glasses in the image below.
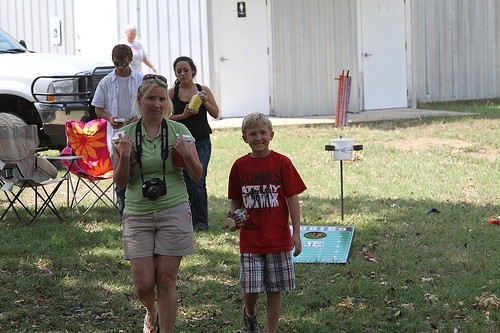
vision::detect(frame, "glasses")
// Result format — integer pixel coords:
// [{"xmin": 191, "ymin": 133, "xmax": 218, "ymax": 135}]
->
[
  {"xmin": 142, "ymin": 74, "xmax": 167, "ymax": 83},
  {"xmin": 114, "ymin": 61, "xmax": 129, "ymax": 67}
]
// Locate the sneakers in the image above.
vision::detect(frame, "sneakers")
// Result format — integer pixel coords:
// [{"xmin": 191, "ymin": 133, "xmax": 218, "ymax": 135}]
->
[
  {"xmin": 242, "ymin": 305, "xmax": 261, "ymax": 333},
  {"xmin": 143, "ymin": 300, "xmax": 160, "ymax": 333}
]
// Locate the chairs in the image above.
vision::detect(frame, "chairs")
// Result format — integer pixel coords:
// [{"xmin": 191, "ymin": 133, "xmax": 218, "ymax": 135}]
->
[
  {"xmin": 0, "ymin": 123, "xmax": 83, "ymax": 227},
  {"xmin": 60, "ymin": 117, "xmax": 118, "ymax": 216}
]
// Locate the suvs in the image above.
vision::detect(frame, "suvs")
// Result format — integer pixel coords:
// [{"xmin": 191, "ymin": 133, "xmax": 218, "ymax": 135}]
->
[{"xmin": 0, "ymin": 28, "xmax": 116, "ymax": 147}]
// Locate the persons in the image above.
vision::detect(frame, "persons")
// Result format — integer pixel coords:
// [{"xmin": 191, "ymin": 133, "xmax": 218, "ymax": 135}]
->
[
  {"xmin": 121, "ymin": 26, "xmax": 157, "ymax": 74},
  {"xmin": 168, "ymin": 57, "xmax": 219, "ymax": 233},
  {"xmin": 228, "ymin": 112, "xmax": 307, "ymax": 333},
  {"xmin": 113, "ymin": 74, "xmax": 203, "ymax": 333},
  {"xmin": 91, "ymin": 44, "xmax": 145, "ymax": 221}
]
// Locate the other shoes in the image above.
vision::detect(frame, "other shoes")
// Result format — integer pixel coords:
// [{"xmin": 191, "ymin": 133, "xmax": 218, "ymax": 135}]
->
[{"xmin": 194, "ymin": 222, "xmax": 208, "ymax": 233}]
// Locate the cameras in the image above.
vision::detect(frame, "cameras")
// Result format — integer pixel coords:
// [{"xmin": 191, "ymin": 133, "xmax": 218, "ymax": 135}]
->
[{"xmin": 141, "ymin": 177, "xmax": 167, "ymax": 201}]
